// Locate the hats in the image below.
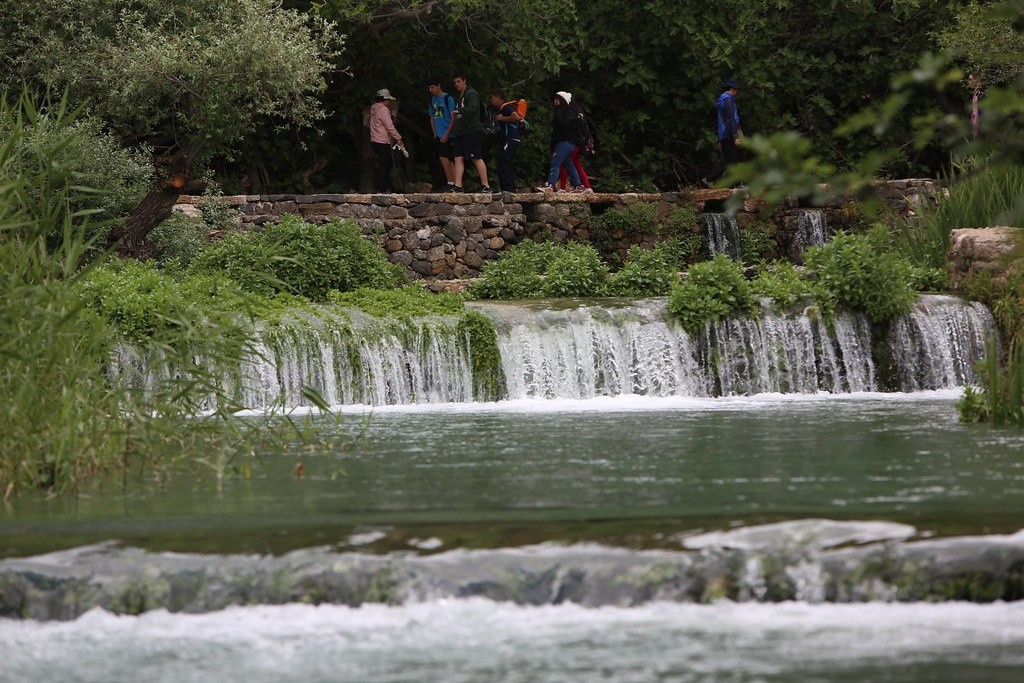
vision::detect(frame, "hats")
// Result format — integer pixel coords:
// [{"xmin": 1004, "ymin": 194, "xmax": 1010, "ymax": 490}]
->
[
  {"xmin": 376, "ymin": 89, "xmax": 396, "ymax": 100},
  {"xmin": 720, "ymin": 80, "xmax": 745, "ymax": 89},
  {"xmin": 556, "ymin": 91, "xmax": 572, "ymax": 105}
]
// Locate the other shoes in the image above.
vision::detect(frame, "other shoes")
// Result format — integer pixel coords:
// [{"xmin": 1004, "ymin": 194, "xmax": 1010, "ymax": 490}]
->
[
  {"xmin": 440, "ymin": 184, "xmax": 455, "ymax": 192},
  {"xmin": 382, "ymin": 189, "xmax": 397, "ymax": 194}
]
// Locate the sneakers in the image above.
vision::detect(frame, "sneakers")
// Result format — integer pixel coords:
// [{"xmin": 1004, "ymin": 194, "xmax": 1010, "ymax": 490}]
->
[
  {"xmin": 569, "ymin": 188, "xmax": 583, "ymax": 193},
  {"xmin": 481, "ymin": 185, "xmax": 492, "ymax": 193},
  {"xmin": 700, "ymin": 177, "xmax": 715, "ymax": 189},
  {"xmin": 583, "ymin": 188, "xmax": 594, "ymax": 194},
  {"xmin": 556, "ymin": 189, "xmax": 566, "ymax": 193},
  {"xmin": 536, "ymin": 186, "xmax": 554, "ymax": 194},
  {"xmin": 450, "ymin": 186, "xmax": 463, "ymax": 192}
]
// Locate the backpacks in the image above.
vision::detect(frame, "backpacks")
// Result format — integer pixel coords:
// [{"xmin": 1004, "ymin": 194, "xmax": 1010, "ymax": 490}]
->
[
  {"xmin": 429, "ymin": 94, "xmax": 458, "ymax": 112},
  {"xmin": 499, "ymin": 98, "xmax": 528, "ymax": 129},
  {"xmin": 466, "ymin": 89, "xmax": 496, "ymax": 137},
  {"xmin": 571, "ymin": 109, "xmax": 595, "ymax": 161}
]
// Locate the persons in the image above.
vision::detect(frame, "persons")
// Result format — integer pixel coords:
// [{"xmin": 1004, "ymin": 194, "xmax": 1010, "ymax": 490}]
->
[
  {"xmin": 428, "ymin": 76, "xmax": 455, "ymax": 194},
  {"xmin": 535, "ymin": 92, "xmax": 593, "ymax": 192},
  {"xmin": 490, "ymin": 90, "xmax": 521, "ymax": 194},
  {"xmin": 371, "ymin": 89, "xmax": 404, "ymax": 193},
  {"xmin": 450, "ymin": 74, "xmax": 493, "ymax": 193},
  {"xmin": 703, "ymin": 81, "xmax": 741, "ymax": 188}
]
